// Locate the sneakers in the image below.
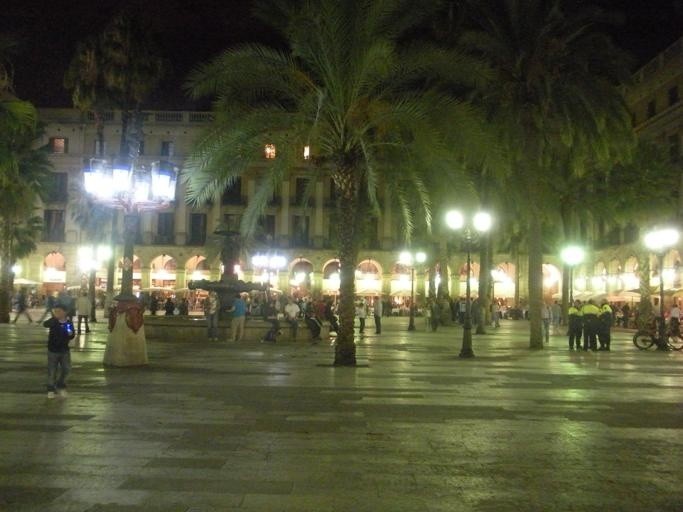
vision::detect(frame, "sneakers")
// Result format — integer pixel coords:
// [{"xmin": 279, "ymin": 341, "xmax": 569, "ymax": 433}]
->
[{"xmin": 48, "ymin": 391, "xmax": 54, "ymax": 399}]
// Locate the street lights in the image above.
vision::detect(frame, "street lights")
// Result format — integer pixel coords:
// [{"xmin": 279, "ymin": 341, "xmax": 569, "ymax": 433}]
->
[
  {"xmin": 446, "ymin": 210, "xmax": 496, "ymax": 358},
  {"xmin": 397, "ymin": 250, "xmax": 428, "ymax": 332},
  {"xmin": 561, "ymin": 244, "xmax": 585, "ymax": 308},
  {"xmin": 250, "ymin": 253, "xmax": 286, "ymax": 321},
  {"xmin": 644, "ymin": 225, "xmax": 679, "ymax": 335},
  {"xmin": 73, "ymin": 241, "xmax": 111, "ymax": 321},
  {"xmin": 82, "ymin": 135, "xmax": 179, "ymax": 304}
]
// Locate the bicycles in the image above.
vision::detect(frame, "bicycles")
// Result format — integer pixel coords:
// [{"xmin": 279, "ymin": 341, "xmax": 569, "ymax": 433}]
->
[{"xmin": 633, "ymin": 317, "xmax": 682, "ymax": 351}]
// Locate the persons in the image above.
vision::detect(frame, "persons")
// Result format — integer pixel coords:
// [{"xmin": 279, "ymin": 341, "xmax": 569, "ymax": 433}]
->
[
  {"xmin": 137, "ymin": 290, "xmax": 338, "ymax": 342},
  {"xmin": 10, "ymin": 287, "xmax": 105, "ymax": 335},
  {"xmin": 359, "ymin": 296, "xmax": 529, "ymax": 332},
  {"xmin": 44, "ymin": 305, "xmax": 75, "ymax": 399},
  {"xmin": 540, "ymin": 297, "xmax": 683, "ymax": 351}
]
